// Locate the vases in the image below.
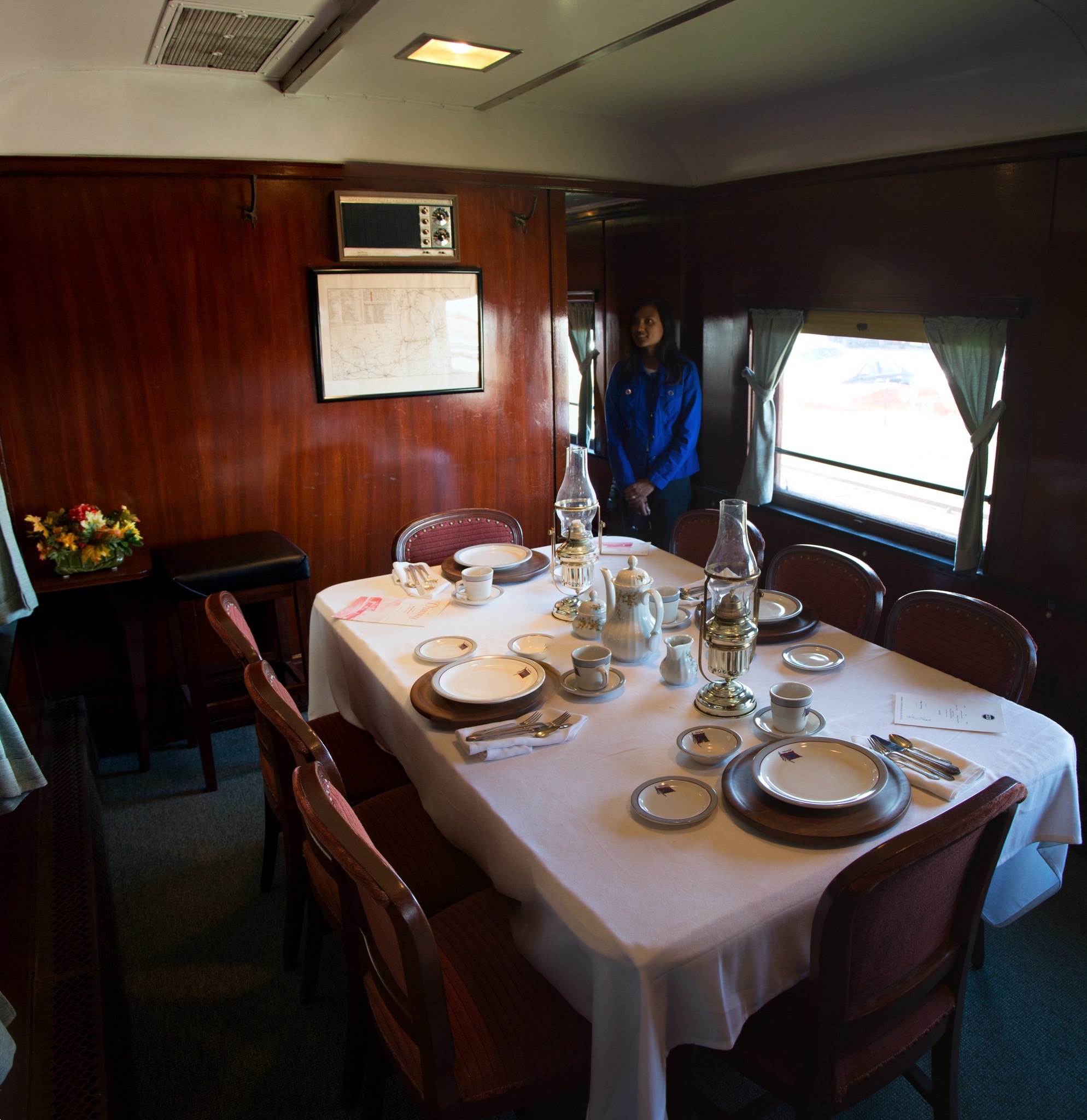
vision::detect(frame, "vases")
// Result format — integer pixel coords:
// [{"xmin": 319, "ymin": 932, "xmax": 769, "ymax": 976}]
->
[{"xmin": 53, "ymin": 549, "xmax": 125, "ymax": 579}]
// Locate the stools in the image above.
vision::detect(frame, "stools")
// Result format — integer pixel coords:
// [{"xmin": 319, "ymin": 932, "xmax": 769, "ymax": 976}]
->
[{"xmin": 151, "ymin": 529, "xmax": 310, "ymax": 791}]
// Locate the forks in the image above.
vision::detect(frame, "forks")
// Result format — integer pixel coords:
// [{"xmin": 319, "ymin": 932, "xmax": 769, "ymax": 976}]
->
[
  {"xmin": 414, "ymin": 565, "xmax": 438, "ymax": 590},
  {"xmin": 867, "ymin": 735, "xmax": 955, "ymax": 780},
  {"xmin": 680, "ymin": 582, "xmax": 744, "ymax": 600}
]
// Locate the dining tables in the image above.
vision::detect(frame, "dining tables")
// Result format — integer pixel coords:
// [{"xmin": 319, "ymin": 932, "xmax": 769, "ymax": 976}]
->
[{"xmin": 307, "ymin": 535, "xmax": 1083, "ymax": 1119}]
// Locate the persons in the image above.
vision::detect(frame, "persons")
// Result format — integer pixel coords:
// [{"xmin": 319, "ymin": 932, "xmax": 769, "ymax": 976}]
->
[{"xmin": 604, "ymin": 295, "xmax": 702, "ymax": 553}]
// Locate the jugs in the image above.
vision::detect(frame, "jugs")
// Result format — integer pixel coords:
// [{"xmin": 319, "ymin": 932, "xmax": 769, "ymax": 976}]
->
[{"xmin": 659, "ymin": 634, "xmax": 698, "ymax": 685}]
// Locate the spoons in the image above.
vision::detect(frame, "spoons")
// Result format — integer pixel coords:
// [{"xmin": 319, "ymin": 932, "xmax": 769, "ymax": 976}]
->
[
  {"xmin": 404, "ymin": 566, "xmax": 416, "ymax": 588},
  {"xmin": 676, "ymin": 579, "xmax": 727, "ymax": 593},
  {"xmin": 888, "ymin": 734, "xmax": 952, "ymax": 765}
]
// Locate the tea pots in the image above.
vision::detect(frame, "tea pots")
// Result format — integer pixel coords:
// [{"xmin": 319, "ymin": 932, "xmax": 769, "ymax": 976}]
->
[{"xmin": 600, "ymin": 555, "xmax": 664, "ymax": 664}]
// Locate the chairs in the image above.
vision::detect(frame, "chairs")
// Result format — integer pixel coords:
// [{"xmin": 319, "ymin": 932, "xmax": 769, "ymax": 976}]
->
[
  {"xmin": 668, "ymin": 509, "xmax": 766, "ymax": 585},
  {"xmin": 247, "ymin": 659, "xmax": 491, "ymax": 1120},
  {"xmin": 390, "ymin": 508, "xmax": 526, "ymax": 571},
  {"xmin": 205, "ymin": 591, "xmax": 408, "ymax": 978},
  {"xmin": 767, "ymin": 544, "xmax": 886, "ymax": 644},
  {"xmin": 683, "ymin": 777, "xmax": 1028, "ymax": 1120},
  {"xmin": 294, "ymin": 760, "xmax": 593, "ymax": 1119},
  {"xmin": 882, "ymin": 589, "xmax": 1036, "ymax": 706}
]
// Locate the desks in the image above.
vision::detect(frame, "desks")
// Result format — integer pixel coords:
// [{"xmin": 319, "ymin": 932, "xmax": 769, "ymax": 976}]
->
[{"xmin": 19, "ymin": 549, "xmax": 152, "ymax": 770}]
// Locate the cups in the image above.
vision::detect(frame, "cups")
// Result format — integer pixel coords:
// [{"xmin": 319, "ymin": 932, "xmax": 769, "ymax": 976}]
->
[
  {"xmin": 769, "ymin": 682, "xmax": 814, "ymax": 733},
  {"xmin": 571, "ymin": 645, "xmax": 611, "ymax": 690},
  {"xmin": 649, "ymin": 586, "xmax": 680, "ymax": 624},
  {"xmin": 454, "ymin": 566, "xmax": 494, "ymax": 601}
]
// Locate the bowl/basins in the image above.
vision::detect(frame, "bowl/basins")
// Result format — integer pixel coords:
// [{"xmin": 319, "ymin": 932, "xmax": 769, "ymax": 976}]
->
[
  {"xmin": 550, "ymin": 564, "xmax": 562, "ymax": 582},
  {"xmin": 507, "ymin": 633, "xmax": 554, "ymax": 659},
  {"xmin": 572, "ymin": 590, "xmax": 606, "ymax": 639},
  {"xmin": 676, "ymin": 725, "xmax": 743, "ymax": 766}
]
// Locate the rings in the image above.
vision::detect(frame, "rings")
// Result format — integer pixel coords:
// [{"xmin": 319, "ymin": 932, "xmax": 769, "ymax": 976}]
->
[
  {"xmin": 631, "ymin": 495, "xmax": 636, "ymax": 500},
  {"xmin": 645, "ymin": 501, "xmax": 648, "ymax": 504}
]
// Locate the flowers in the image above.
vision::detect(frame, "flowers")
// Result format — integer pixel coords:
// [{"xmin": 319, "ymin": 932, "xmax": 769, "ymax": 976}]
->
[{"xmin": 22, "ymin": 503, "xmax": 145, "ymax": 564}]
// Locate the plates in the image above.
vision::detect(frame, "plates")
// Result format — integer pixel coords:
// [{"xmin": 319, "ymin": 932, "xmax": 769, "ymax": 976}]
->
[
  {"xmin": 753, "ymin": 705, "xmax": 826, "ymax": 739},
  {"xmin": 431, "ymin": 655, "xmax": 546, "ymax": 703},
  {"xmin": 736, "ymin": 589, "xmax": 803, "ymax": 624},
  {"xmin": 782, "ymin": 644, "xmax": 846, "ymax": 672},
  {"xmin": 751, "ymin": 736, "xmax": 888, "ymax": 809},
  {"xmin": 414, "ymin": 636, "xmax": 477, "ymax": 661},
  {"xmin": 453, "ymin": 543, "xmax": 532, "ymax": 571},
  {"xmin": 561, "ymin": 667, "xmax": 626, "ymax": 698},
  {"xmin": 451, "ymin": 584, "xmax": 505, "ymax": 606},
  {"xmin": 663, "ymin": 607, "xmax": 691, "ymax": 628},
  {"xmin": 631, "ymin": 775, "xmax": 718, "ymax": 825}
]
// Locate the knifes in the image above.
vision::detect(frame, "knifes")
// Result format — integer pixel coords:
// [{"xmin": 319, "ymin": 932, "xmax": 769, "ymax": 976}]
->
[
  {"xmin": 408, "ymin": 565, "xmax": 424, "ymax": 596},
  {"xmin": 870, "ymin": 734, "xmax": 961, "ymax": 774}
]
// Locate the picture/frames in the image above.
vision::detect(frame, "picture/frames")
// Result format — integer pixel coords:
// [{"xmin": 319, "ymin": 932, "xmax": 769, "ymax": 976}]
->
[{"xmin": 307, "ymin": 265, "xmax": 484, "ymax": 403}]
